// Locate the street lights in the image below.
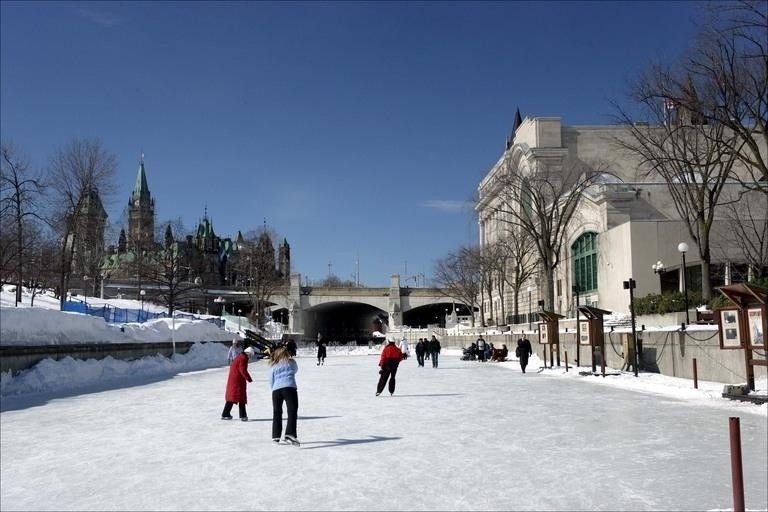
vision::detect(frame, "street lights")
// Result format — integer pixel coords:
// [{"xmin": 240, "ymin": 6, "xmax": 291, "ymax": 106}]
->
[
  {"xmin": 652, "ymin": 260, "xmax": 667, "ymax": 296},
  {"xmin": 526, "ymin": 286, "xmax": 532, "ymax": 331},
  {"xmin": 213, "ymin": 295, "xmax": 226, "ymax": 317},
  {"xmin": 455, "ymin": 307, "xmax": 460, "ymax": 332},
  {"xmin": 255, "ymin": 313, "xmax": 260, "ymax": 331},
  {"xmin": 571, "ymin": 284, "xmax": 585, "ymax": 368},
  {"xmin": 82, "ymin": 276, "xmax": 88, "ymax": 304},
  {"xmin": 279, "ymin": 312, "xmax": 284, "ymax": 325},
  {"xmin": 237, "ymin": 309, "xmax": 242, "ymax": 331},
  {"xmin": 140, "ymin": 289, "xmax": 146, "ymax": 314},
  {"xmin": 622, "ymin": 279, "xmax": 640, "ymax": 377},
  {"xmin": 444, "ymin": 308, "xmax": 448, "ymax": 330},
  {"xmin": 535, "ymin": 300, "xmax": 548, "ymax": 368},
  {"xmin": 677, "ymin": 242, "xmax": 690, "ymax": 326}
]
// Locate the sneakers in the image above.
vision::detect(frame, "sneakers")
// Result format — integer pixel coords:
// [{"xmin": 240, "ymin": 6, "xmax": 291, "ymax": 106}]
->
[
  {"xmin": 285, "ymin": 435, "xmax": 301, "ymax": 445},
  {"xmin": 272, "ymin": 438, "xmax": 279, "ymax": 442}
]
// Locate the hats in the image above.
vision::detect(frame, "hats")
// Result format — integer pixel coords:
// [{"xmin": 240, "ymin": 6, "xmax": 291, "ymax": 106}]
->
[
  {"xmin": 389, "ymin": 337, "xmax": 395, "ymax": 342},
  {"xmin": 244, "ymin": 346, "xmax": 255, "ymax": 354}
]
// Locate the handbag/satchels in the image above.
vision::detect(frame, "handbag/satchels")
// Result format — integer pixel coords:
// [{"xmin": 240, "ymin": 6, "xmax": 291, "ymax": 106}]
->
[{"xmin": 516, "ymin": 347, "xmax": 520, "ymax": 357}]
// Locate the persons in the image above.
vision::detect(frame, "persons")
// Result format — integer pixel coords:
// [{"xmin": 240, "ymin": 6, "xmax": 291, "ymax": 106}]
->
[
  {"xmin": 316, "ymin": 331, "xmax": 326, "ymax": 366},
  {"xmin": 461, "ymin": 335, "xmax": 508, "ymax": 362},
  {"xmin": 227, "ymin": 339, "xmax": 297, "ymax": 369},
  {"xmin": 222, "ymin": 347, "xmax": 254, "ymax": 421},
  {"xmin": 399, "ymin": 335, "xmax": 441, "ymax": 368},
  {"xmin": 268, "ymin": 347, "xmax": 300, "ymax": 445},
  {"xmin": 377, "ymin": 336, "xmax": 403, "ymax": 394},
  {"xmin": 517, "ymin": 334, "xmax": 532, "ymax": 373}
]
